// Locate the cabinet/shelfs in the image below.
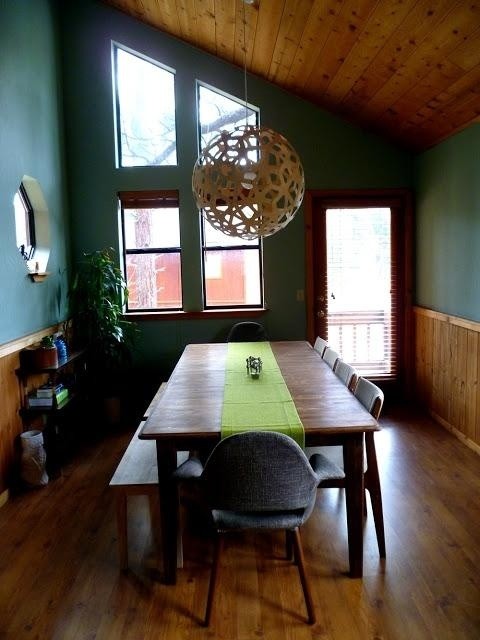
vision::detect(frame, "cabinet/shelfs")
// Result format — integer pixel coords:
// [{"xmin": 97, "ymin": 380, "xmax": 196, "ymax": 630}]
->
[{"xmin": 15, "ymin": 350, "xmax": 86, "ymax": 480}]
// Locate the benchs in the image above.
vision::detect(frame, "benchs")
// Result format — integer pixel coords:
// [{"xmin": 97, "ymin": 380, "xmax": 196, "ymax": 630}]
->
[
  {"xmin": 143, "ymin": 382, "xmax": 167, "ymax": 421},
  {"xmin": 109, "ymin": 420, "xmax": 190, "ymax": 570}
]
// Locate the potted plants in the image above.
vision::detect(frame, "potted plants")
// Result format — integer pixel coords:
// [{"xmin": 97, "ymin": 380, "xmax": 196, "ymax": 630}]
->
[{"xmin": 71, "ymin": 247, "xmax": 144, "ymax": 426}]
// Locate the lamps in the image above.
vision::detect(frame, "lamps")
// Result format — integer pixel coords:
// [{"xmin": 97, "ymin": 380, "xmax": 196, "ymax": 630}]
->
[{"xmin": 192, "ymin": 0, "xmax": 305, "ymax": 240}]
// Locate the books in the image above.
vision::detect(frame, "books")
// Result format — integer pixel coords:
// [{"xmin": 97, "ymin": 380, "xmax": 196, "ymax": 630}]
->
[{"xmin": 26, "ymin": 381, "xmax": 70, "ymax": 410}]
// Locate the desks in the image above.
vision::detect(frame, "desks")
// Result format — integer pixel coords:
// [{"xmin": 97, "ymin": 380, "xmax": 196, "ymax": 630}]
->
[{"xmin": 138, "ymin": 341, "xmax": 379, "ymax": 585}]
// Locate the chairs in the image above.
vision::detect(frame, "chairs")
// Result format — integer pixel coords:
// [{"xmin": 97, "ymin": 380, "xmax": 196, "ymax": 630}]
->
[
  {"xmin": 285, "ymin": 336, "xmax": 387, "ymax": 562},
  {"xmin": 226, "ymin": 321, "xmax": 270, "ymax": 343},
  {"xmin": 171, "ymin": 431, "xmax": 345, "ymax": 628}
]
